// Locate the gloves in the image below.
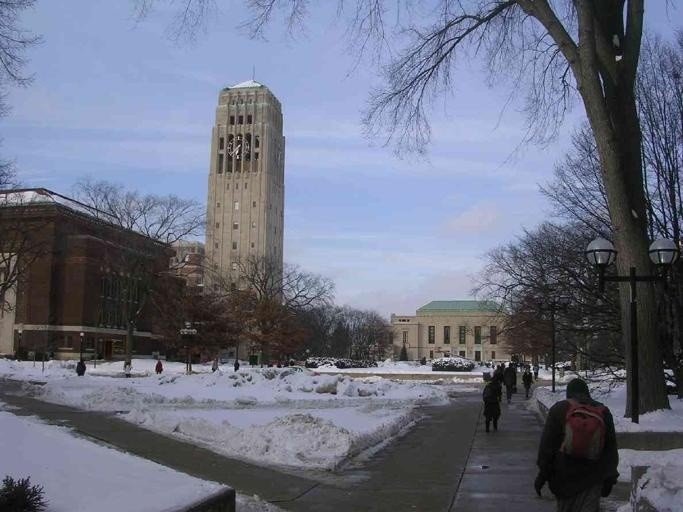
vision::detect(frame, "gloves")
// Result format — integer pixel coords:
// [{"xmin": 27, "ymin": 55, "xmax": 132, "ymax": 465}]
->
[
  {"xmin": 601, "ymin": 480, "xmax": 613, "ymax": 497},
  {"xmin": 534, "ymin": 473, "xmax": 545, "ymax": 496}
]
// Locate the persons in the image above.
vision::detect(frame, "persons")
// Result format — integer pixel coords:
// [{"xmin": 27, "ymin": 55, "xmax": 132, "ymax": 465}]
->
[
  {"xmin": 233, "ymin": 359, "xmax": 239, "ymax": 372},
  {"xmin": 122, "ymin": 353, "xmax": 132, "ymax": 370},
  {"xmin": 211, "ymin": 359, "xmax": 218, "ymax": 373},
  {"xmin": 124, "ymin": 362, "xmax": 132, "ymax": 378},
  {"xmin": 534, "ymin": 377, "xmax": 620, "ymax": 511},
  {"xmin": 155, "ymin": 360, "xmax": 163, "ymax": 374},
  {"xmin": 75, "ymin": 359, "xmax": 86, "ymax": 376},
  {"xmin": 482, "ymin": 376, "xmax": 501, "ymax": 432},
  {"xmin": 492, "ymin": 358, "xmax": 539, "ymax": 403}
]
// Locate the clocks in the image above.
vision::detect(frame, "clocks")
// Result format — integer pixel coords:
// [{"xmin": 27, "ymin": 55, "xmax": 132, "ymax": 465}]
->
[{"xmin": 227, "ymin": 135, "xmax": 249, "ymax": 160}]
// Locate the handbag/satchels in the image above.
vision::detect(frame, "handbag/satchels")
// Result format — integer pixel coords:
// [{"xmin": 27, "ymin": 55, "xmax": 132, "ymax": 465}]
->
[{"xmin": 511, "ymin": 388, "xmax": 517, "ymax": 393}]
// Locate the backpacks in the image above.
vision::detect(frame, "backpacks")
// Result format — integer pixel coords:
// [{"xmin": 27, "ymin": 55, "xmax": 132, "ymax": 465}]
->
[{"xmin": 556, "ymin": 398, "xmax": 607, "ymax": 463}]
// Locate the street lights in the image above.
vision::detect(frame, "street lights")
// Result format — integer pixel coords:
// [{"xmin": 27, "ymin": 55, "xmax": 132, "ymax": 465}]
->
[
  {"xmin": 584, "ymin": 235, "xmax": 679, "ymax": 424},
  {"xmin": 179, "ymin": 321, "xmax": 198, "ymax": 372},
  {"xmin": 79, "ymin": 332, "xmax": 85, "ymax": 362},
  {"xmin": 18, "ymin": 329, "xmax": 22, "ymax": 361},
  {"xmin": 533, "ymin": 292, "xmax": 573, "ymax": 393}
]
